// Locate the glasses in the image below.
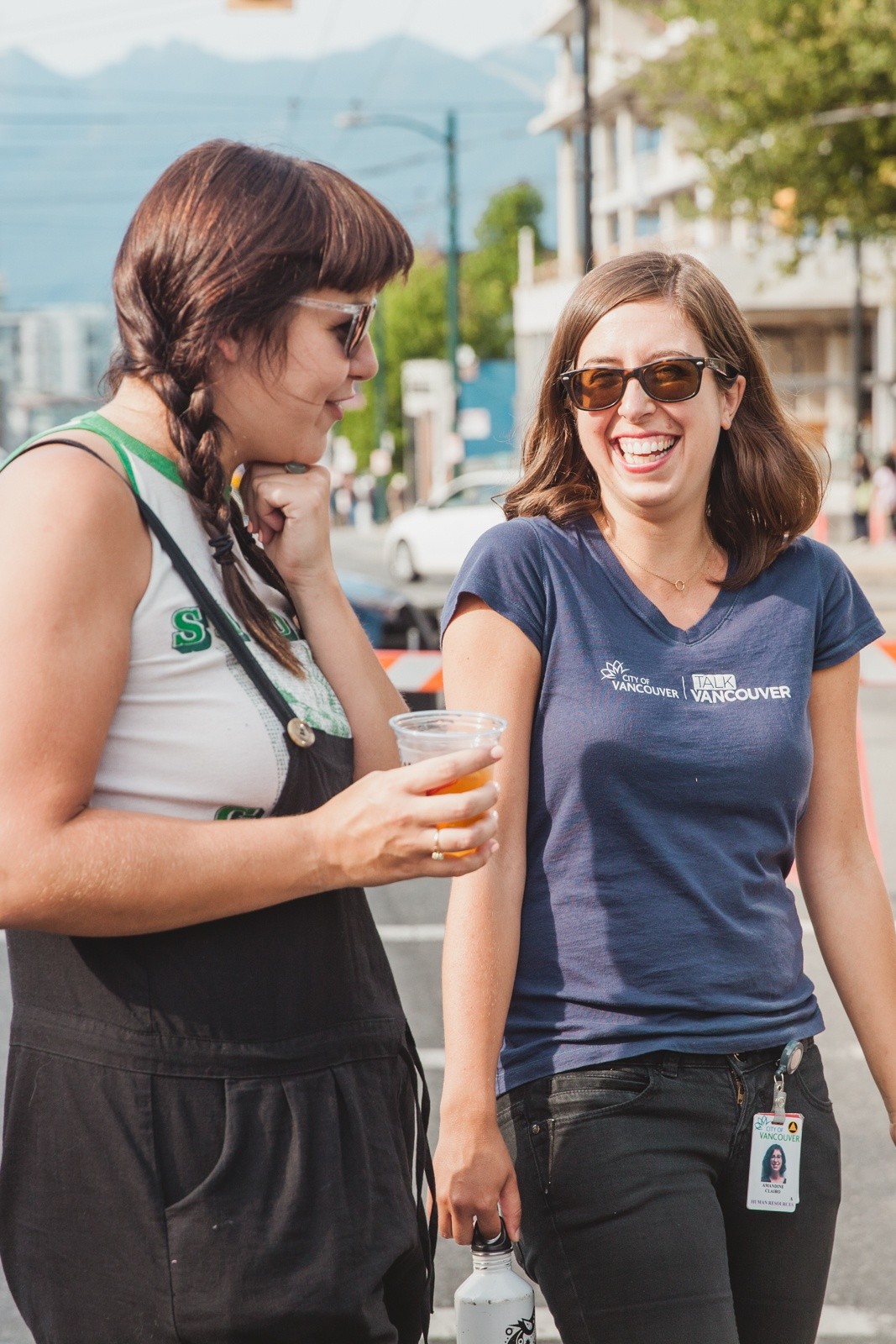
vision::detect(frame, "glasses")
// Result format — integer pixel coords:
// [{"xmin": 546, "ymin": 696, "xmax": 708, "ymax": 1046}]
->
[
  {"xmin": 557, "ymin": 355, "xmax": 740, "ymax": 412},
  {"xmin": 286, "ymin": 295, "xmax": 378, "ymax": 358}
]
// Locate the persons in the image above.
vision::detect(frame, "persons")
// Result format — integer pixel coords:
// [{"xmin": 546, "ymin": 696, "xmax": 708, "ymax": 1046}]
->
[
  {"xmin": 0, "ymin": 138, "xmax": 498, "ymax": 1344},
  {"xmin": 427, "ymin": 250, "xmax": 896, "ymax": 1344},
  {"xmin": 761, "ymin": 1144, "xmax": 788, "ymax": 1183}
]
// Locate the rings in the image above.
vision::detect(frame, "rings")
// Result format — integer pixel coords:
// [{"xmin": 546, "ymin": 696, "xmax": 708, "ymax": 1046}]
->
[{"xmin": 284, "ymin": 463, "xmax": 308, "ymax": 473}]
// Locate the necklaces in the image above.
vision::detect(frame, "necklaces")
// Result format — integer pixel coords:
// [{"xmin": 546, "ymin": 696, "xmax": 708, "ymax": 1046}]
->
[{"xmin": 593, "ymin": 507, "xmax": 713, "ymax": 593}]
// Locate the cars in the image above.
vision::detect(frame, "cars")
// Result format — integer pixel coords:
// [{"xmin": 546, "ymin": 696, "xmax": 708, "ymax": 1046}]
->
[
  {"xmin": 379, "ymin": 467, "xmax": 526, "ymax": 585},
  {"xmin": 339, "ymin": 567, "xmax": 443, "ymax": 711}
]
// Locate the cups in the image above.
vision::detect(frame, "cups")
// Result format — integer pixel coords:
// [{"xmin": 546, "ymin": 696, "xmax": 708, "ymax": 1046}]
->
[{"xmin": 389, "ymin": 708, "xmax": 509, "ymax": 861}]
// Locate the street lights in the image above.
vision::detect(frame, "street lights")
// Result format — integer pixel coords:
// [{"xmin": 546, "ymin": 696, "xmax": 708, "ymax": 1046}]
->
[{"xmin": 336, "ymin": 102, "xmax": 465, "ymax": 507}]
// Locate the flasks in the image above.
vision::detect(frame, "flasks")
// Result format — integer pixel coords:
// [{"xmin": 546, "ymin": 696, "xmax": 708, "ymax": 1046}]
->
[{"xmin": 453, "ymin": 1216, "xmax": 537, "ymax": 1344}]
